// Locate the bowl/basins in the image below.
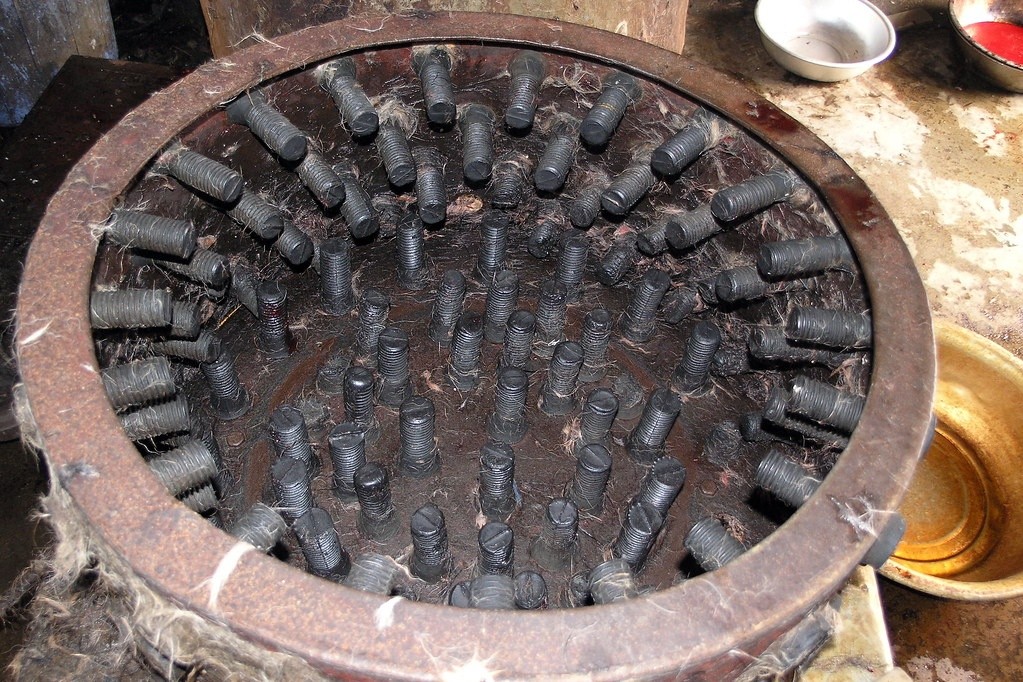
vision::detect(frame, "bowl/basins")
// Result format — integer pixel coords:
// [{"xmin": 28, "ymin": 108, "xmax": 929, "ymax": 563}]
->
[
  {"xmin": 755, "ymin": 0, "xmax": 897, "ymax": 83},
  {"xmin": 946, "ymin": 0, "xmax": 1023, "ymax": 94},
  {"xmin": 875, "ymin": 316, "xmax": 1023, "ymax": 600}
]
